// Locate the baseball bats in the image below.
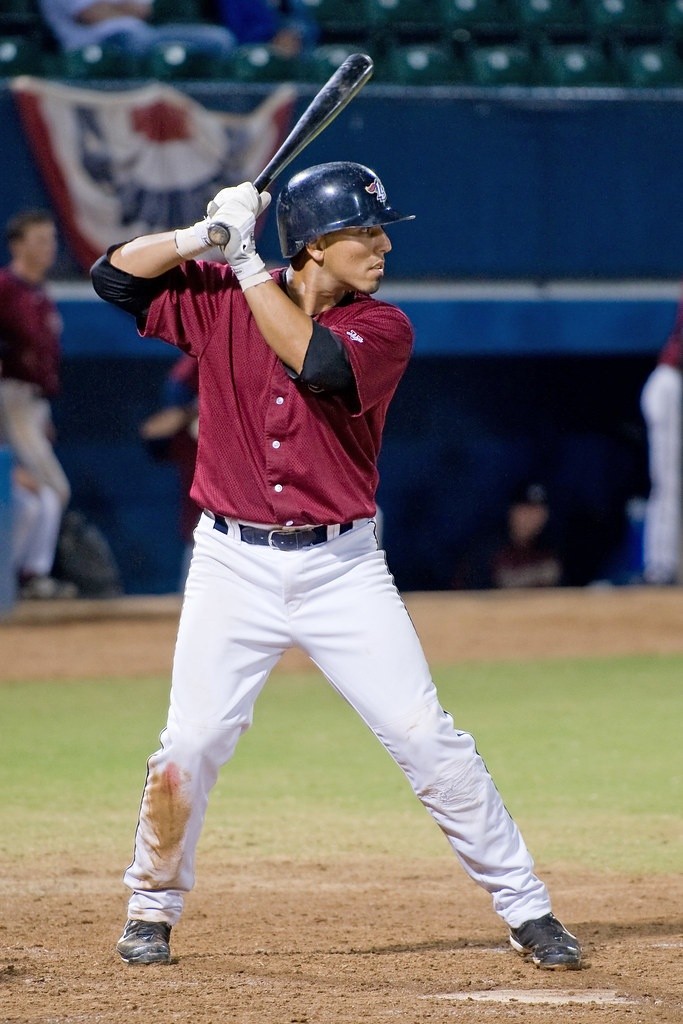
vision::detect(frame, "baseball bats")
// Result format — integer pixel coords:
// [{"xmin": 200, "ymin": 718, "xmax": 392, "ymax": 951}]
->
[{"xmin": 208, "ymin": 53, "xmax": 374, "ymax": 246}]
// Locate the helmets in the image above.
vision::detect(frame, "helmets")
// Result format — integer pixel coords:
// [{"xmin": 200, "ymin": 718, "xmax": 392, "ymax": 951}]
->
[{"xmin": 276, "ymin": 161, "xmax": 415, "ymax": 259}]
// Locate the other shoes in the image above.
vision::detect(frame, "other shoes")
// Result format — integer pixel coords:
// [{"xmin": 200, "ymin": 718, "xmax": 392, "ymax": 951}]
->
[{"xmin": 18, "ymin": 568, "xmax": 77, "ymax": 603}]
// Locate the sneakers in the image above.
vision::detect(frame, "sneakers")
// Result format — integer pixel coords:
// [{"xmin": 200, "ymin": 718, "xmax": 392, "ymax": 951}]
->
[
  {"xmin": 116, "ymin": 919, "xmax": 172, "ymax": 965},
  {"xmin": 509, "ymin": 912, "xmax": 582, "ymax": 970}
]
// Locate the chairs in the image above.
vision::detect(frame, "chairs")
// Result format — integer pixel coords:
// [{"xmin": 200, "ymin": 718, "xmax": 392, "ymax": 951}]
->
[{"xmin": 0, "ymin": 0, "xmax": 683, "ymax": 89}]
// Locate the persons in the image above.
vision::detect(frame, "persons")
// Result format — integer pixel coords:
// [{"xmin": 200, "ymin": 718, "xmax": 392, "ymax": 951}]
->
[
  {"xmin": 0, "ymin": 208, "xmax": 80, "ymax": 599},
  {"xmin": 489, "ymin": 487, "xmax": 561, "ymax": 589},
  {"xmin": 639, "ymin": 309, "xmax": 683, "ymax": 581},
  {"xmin": 142, "ymin": 392, "xmax": 203, "ymax": 595},
  {"xmin": 90, "ymin": 161, "xmax": 585, "ymax": 970},
  {"xmin": 33, "ymin": 0, "xmax": 320, "ymax": 76}
]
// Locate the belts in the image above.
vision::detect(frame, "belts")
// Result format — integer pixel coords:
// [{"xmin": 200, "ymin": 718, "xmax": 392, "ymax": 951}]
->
[{"xmin": 214, "ymin": 511, "xmax": 353, "ymax": 552}]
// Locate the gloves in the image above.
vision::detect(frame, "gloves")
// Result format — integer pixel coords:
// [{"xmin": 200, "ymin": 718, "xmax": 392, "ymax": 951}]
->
[
  {"xmin": 206, "ymin": 181, "xmax": 266, "ymax": 281},
  {"xmin": 192, "ymin": 189, "xmax": 271, "ymax": 249}
]
